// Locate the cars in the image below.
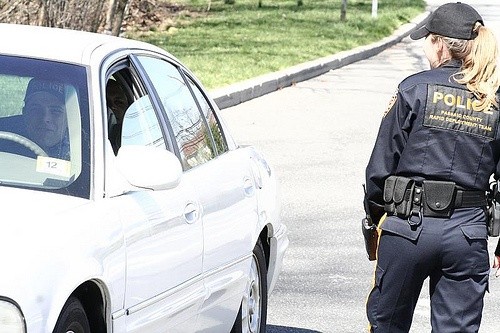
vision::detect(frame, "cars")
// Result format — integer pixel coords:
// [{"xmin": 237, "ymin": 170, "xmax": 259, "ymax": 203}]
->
[{"xmin": 0, "ymin": 23, "xmax": 291, "ymax": 333}]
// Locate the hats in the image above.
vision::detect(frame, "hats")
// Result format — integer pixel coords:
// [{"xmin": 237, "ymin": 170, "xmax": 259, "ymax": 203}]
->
[
  {"xmin": 24, "ymin": 77, "xmax": 65, "ymax": 104},
  {"xmin": 410, "ymin": 2, "xmax": 484, "ymax": 41}
]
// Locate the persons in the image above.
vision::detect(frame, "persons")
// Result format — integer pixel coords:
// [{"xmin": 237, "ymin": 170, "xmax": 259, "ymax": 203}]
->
[
  {"xmin": 106, "ymin": 80, "xmax": 133, "ymax": 156},
  {"xmin": 362, "ymin": 1, "xmax": 500, "ymax": 333},
  {"xmin": 21, "ymin": 77, "xmax": 91, "ymax": 200}
]
